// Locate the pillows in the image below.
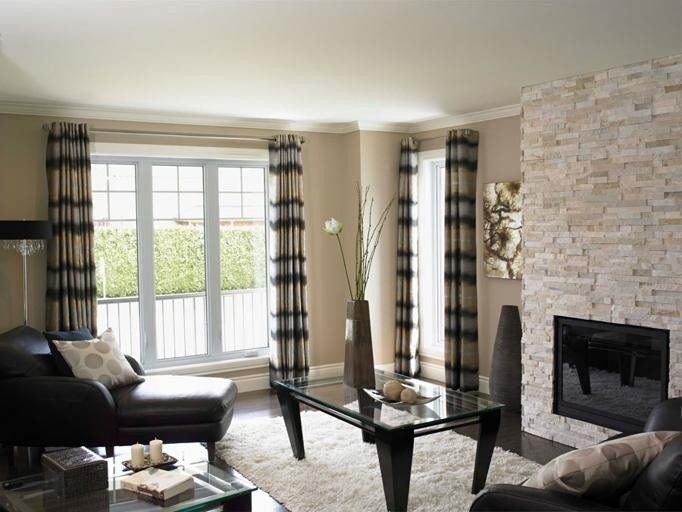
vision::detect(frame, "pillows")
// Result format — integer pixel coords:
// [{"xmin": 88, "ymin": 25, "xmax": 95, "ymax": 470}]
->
[
  {"xmin": 53, "ymin": 327, "xmax": 146, "ymax": 390},
  {"xmin": 520, "ymin": 430, "xmax": 682, "ymax": 497},
  {"xmin": 42, "ymin": 328, "xmax": 94, "ymax": 377}
]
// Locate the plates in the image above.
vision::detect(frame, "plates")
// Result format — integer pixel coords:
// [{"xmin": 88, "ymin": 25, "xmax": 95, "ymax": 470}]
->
[
  {"xmin": 123, "ymin": 452, "xmax": 178, "ymax": 471},
  {"xmin": 362, "ymin": 387, "xmax": 442, "ymax": 410}
]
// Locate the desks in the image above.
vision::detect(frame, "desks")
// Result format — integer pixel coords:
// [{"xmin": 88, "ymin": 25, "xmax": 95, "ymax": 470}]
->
[
  {"xmin": 0, "ymin": 457, "xmax": 258, "ymax": 512},
  {"xmin": 273, "ymin": 369, "xmax": 506, "ymax": 512}
]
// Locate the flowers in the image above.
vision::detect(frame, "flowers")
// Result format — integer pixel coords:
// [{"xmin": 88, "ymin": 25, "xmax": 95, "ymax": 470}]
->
[{"xmin": 321, "ymin": 181, "xmax": 397, "ymax": 301}]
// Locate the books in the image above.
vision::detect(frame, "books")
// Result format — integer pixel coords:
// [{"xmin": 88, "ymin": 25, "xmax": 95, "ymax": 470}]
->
[{"xmin": 119, "ymin": 466, "xmax": 195, "ymax": 502}]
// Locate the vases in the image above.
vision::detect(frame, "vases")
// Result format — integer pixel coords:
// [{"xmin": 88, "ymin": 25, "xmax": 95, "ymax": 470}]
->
[{"xmin": 343, "ymin": 302, "xmax": 376, "ymax": 388}]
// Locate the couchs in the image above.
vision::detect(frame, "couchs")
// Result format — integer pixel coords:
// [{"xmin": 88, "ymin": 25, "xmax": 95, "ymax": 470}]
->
[
  {"xmin": 0, "ymin": 324, "xmax": 238, "ymax": 445},
  {"xmin": 468, "ymin": 398, "xmax": 682, "ymax": 511}
]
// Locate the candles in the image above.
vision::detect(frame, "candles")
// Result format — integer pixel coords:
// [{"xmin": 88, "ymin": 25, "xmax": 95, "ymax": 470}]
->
[
  {"xmin": 149, "ymin": 436, "xmax": 163, "ymax": 463},
  {"xmin": 131, "ymin": 441, "xmax": 145, "ymax": 468}
]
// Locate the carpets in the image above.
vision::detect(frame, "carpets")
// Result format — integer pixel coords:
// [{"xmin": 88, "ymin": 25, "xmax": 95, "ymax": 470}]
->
[{"xmin": 199, "ymin": 399, "xmax": 546, "ymax": 512}]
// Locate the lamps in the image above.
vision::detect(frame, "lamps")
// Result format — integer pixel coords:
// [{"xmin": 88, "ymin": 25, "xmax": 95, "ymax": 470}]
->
[{"xmin": 0, "ymin": 218, "xmax": 55, "ymax": 327}]
